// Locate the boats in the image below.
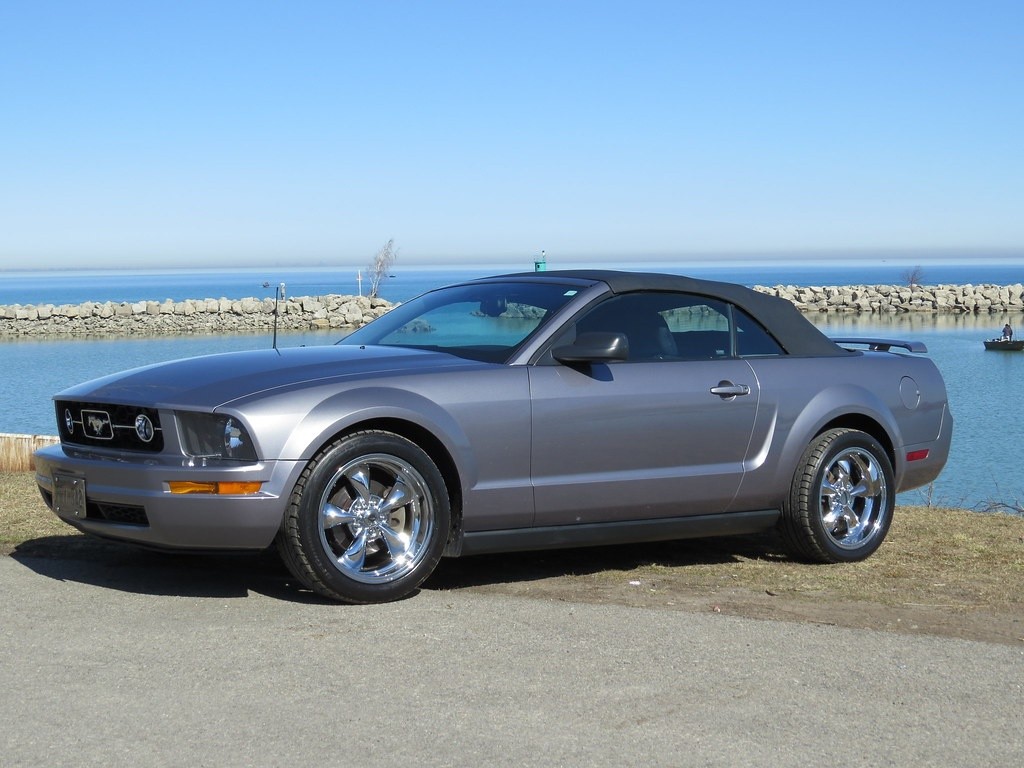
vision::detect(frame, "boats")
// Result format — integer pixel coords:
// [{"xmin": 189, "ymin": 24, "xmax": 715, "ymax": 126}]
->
[{"xmin": 984, "ymin": 340, "xmax": 1024, "ymax": 351}]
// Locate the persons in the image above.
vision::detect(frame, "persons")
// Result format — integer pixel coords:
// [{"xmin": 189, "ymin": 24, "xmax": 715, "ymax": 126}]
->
[{"xmin": 1002, "ymin": 324, "xmax": 1013, "ymax": 341}]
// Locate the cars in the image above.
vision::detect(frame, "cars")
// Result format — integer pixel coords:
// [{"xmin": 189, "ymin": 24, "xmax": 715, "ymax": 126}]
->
[{"xmin": 29, "ymin": 268, "xmax": 953, "ymax": 605}]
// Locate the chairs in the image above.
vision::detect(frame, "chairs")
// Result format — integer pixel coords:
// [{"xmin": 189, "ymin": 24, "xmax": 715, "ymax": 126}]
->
[{"xmin": 628, "ymin": 311, "xmax": 682, "ymax": 356}]
